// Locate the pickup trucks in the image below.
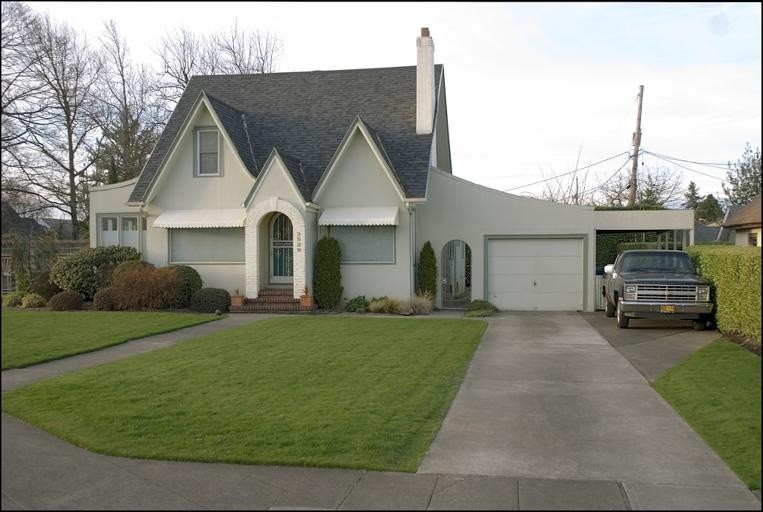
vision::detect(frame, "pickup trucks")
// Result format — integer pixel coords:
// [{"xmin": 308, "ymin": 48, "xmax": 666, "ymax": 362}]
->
[{"xmin": 601, "ymin": 249, "xmax": 714, "ymax": 332}]
